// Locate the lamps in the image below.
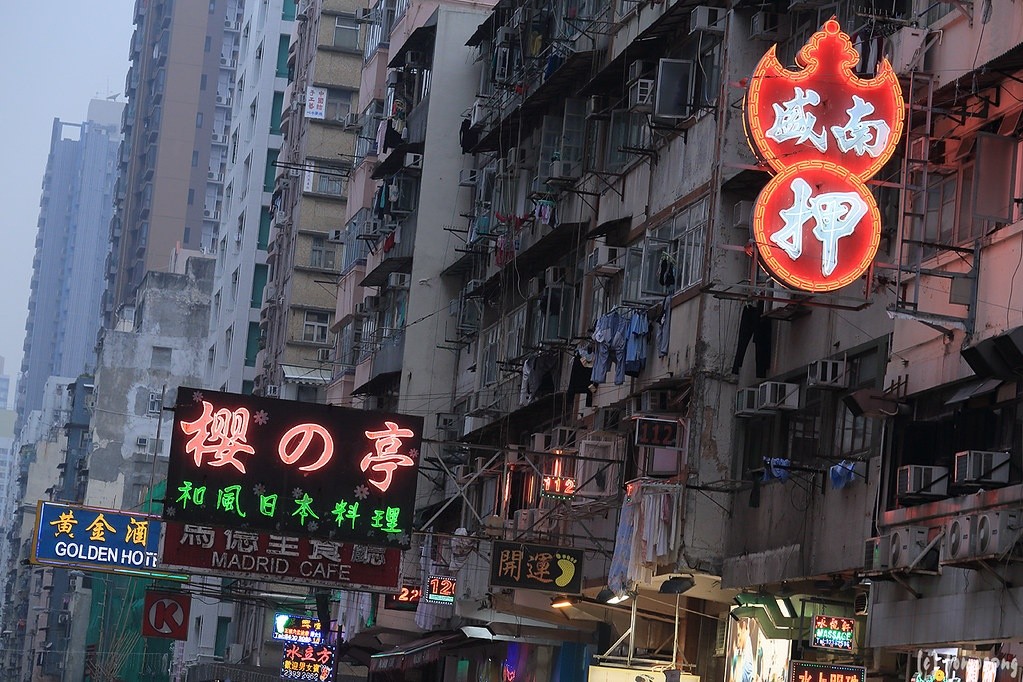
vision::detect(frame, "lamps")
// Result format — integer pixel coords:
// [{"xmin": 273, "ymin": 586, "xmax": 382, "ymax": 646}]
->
[{"xmin": 551, "ymin": 556, "xmax": 694, "ymax": 608}]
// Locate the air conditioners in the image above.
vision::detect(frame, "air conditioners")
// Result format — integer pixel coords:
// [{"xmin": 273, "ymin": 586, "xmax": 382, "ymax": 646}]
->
[
  {"xmin": 763, "ymin": 278, "xmax": 801, "ymax": 319},
  {"xmin": 81, "ymin": 476, "xmax": 88, "ymax": 482},
  {"xmin": 225, "ymin": 645, "xmax": 242, "ymax": 665},
  {"xmin": 137, "ymin": 438, "xmax": 148, "ymax": 445},
  {"xmin": 750, "ymin": 11, "xmax": 787, "ymax": 40},
  {"xmin": 733, "ymin": 201, "xmax": 756, "ymax": 229},
  {"xmin": 203, "ymin": 209, "xmax": 216, "ymax": 219},
  {"xmin": 313, "ymin": 0, "xmax": 725, "ymax": 484},
  {"xmin": 58, "ymin": 615, "xmax": 68, "ymax": 623},
  {"xmin": 84, "ymin": 433, "xmax": 92, "ymax": 439},
  {"xmin": 865, "ymin": 448, "xmax": 1023, "ymax": 582},
  {"xmin": 882, "ymin": 27, "xmax": 927, "ymax": 75},
  {"xmin": 203, "ymin": 20, "xmax": 235, "ymax": 180},
  {"xmin": 504, "ymin": 507, "xmax": 551, "ymax": 541},
  {"xmin": 909, "ymin": 136, "xmax": 958, "ymax": 174},
  {"xmin": 265, "ymin": 384, "xmax": 278, "ymax": 398},
  {"xmin": 70, "ymin": 580, "xmax": 76, "ymax": 586},
  {"xmin": 735, "ymin": 357, "xmax": 849, "ymax": 417}
]
